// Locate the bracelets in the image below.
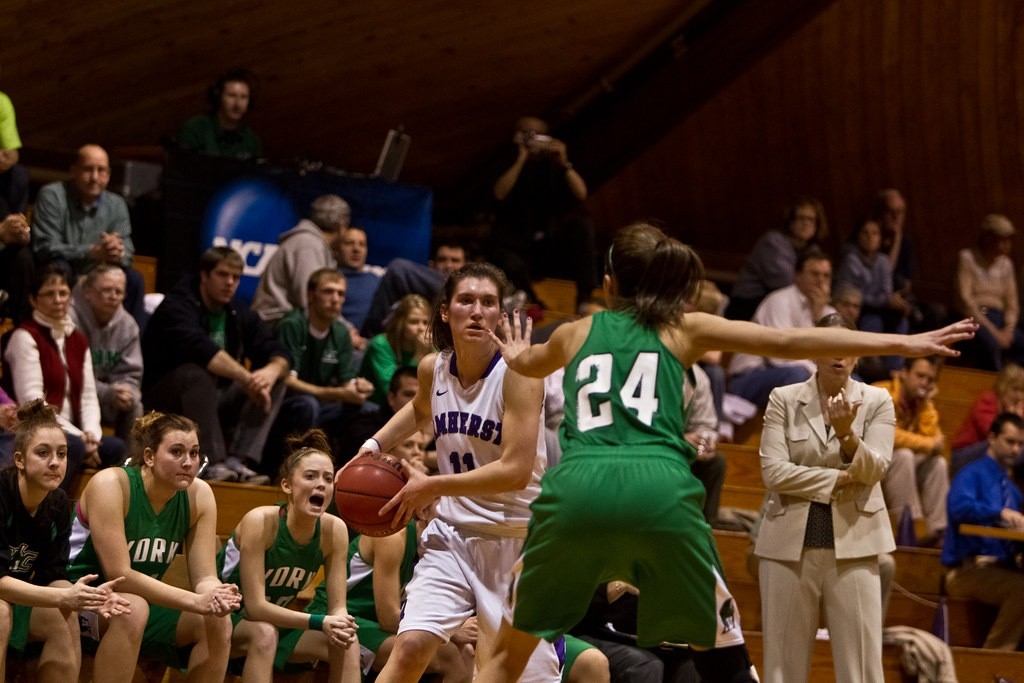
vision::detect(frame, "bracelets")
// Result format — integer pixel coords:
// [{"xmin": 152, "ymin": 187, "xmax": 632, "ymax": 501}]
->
[
  {"xmin": 361, "ymin": 438, "xmax": 381, "ymax": 454},
  {"xmin": 309, "ymin": 613, "xmax": 326, "ymax": 631}
]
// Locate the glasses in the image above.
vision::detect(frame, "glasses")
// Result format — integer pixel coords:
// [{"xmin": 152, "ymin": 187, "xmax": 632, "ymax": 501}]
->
[
  {"xmin": 37, "ymin": 289, "xmax": 71, "ymax": 300},
  {"xmin": 78, "ymin": 165, "xmax": 111, "ymax": 178}
]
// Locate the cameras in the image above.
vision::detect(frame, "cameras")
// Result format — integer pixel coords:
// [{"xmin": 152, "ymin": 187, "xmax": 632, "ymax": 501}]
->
[{"xmin": 524, "ymin": 132, "xmax": 553, "ymax": 146}]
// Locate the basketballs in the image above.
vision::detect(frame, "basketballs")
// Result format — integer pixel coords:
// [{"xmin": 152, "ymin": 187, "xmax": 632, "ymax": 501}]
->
[{"xmin": 336, "ymin": 452, "xmax": 412, "ymax": 538}]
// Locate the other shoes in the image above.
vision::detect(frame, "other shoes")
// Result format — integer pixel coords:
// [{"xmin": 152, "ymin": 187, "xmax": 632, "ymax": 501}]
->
[{"xmin": 209, "ymin": 463, "xmax": 270, "ymax": 485}]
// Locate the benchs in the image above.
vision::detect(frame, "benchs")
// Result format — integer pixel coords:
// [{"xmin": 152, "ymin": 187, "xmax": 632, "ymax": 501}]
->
[{"xmin": 0, "ymin": 161, "xmax": 1024, "ymax": 683}]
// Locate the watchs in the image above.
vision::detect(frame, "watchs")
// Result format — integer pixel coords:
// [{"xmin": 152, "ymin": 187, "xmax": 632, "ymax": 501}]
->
[
  {"xmin": 836, "ymin": 431, "xmax": 854, "ymax": 442},
  {"xmin": 563, "ymin": 163, "xmax": 573, "ymax": 171}
]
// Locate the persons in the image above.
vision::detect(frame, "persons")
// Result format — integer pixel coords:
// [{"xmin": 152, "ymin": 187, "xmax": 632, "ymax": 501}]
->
[{"xmin": 0, "ymin": 65, "xmax": 1024, "ymax": 683}]
